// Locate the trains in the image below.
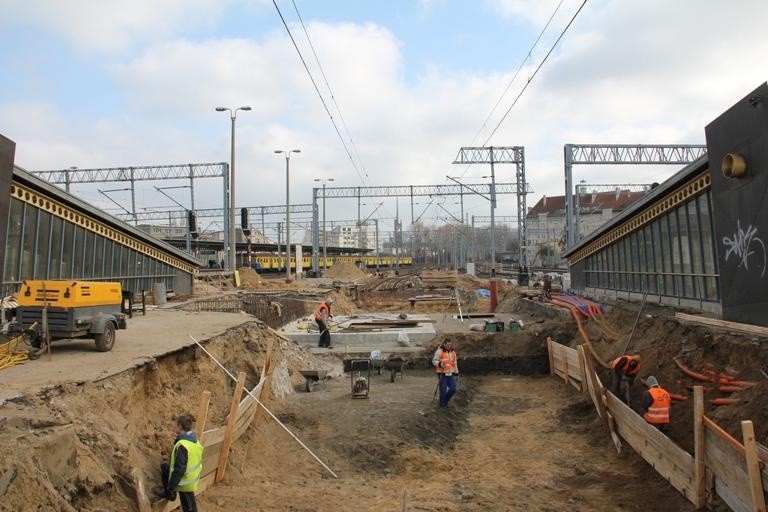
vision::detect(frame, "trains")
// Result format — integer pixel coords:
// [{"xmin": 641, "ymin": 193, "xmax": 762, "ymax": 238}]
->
[{"xmin": 241, "ymin": 252, "xmax": 412, "ymax": 273}]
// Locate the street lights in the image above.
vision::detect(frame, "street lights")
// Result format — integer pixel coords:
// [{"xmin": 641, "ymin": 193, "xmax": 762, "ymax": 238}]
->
[
  {"xmin": 214, "ymin": 106, "xmax": 252, "ymax": 269},
  {"xmin": 273, "ymin": 149, "xmax": 301, "ymax": 279},
  {"xmin": 313, "ymin": 178, "xmax": 335, "ymax": 273}
]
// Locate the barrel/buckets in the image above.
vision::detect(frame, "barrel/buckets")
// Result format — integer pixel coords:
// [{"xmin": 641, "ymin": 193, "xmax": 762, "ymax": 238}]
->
[
  {"xmin": 467, "ymin": 263, "xmax": 475, "ymax": 276},
  {"xmin": 486, "ymin": 321, "xmax": 518, "ymax": 332},
  {"xmin": 153, "ymin": 283, "xmax": 167, "ymax": 304},
  {"xmin": 563, "ymin": 273, "xmax": 571, "ymax": 291}
]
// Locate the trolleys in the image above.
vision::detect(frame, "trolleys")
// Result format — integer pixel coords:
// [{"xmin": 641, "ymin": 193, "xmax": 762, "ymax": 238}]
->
[
  {"xmin": 350, "ymin": 353, "xmax": 410, "ymax": 399},
  {"xmin": 298, "ymin": 367, "xmax": 334, "ymax": 392}
]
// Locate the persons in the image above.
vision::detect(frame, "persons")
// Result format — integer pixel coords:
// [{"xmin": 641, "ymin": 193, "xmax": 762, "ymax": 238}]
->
[
  {"xmin": 153, "ymin": 411, "xmax": 201, "ymax": 511},
  {"xmin": 610, "ymin": 354, "xmax": 641, "ymax": 395},
  {"xmin": 644, "ymin": 375, "xmax": 671, "ymax": 430},
  {"xmin": 315, "ymin": 297, "xmax": 334, "ymax": 348},
  {"xmin": 431, "ymin": 337, "xmax": 458, "ymax": 406}
]
